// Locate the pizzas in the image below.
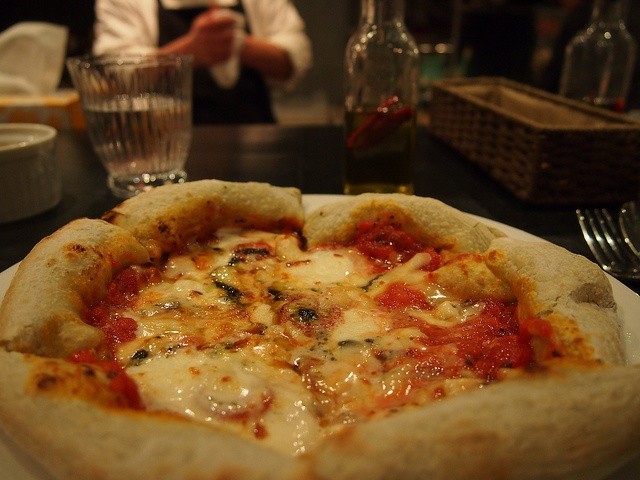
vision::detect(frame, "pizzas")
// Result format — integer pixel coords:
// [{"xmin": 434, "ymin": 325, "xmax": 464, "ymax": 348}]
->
[{"xmin": 0, "ymin": 179, "xmax": 640, "ymax": 479}]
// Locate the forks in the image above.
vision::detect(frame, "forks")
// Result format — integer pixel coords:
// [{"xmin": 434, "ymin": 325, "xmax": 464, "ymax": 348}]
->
[{"xmin": 575, "ymin": 208, "xmax": 639, "ymax": 281}]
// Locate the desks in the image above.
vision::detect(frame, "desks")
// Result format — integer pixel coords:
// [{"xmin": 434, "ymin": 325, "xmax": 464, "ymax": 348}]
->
[{"xmin": 1, "ymin": 122, "xmax": 640, "ymax": 296}]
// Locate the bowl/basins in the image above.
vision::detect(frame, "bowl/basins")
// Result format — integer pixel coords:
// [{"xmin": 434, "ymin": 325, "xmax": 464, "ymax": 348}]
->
[{"xmin": 0, "ymin": 122, "xmax": 62, "ymax": 221}]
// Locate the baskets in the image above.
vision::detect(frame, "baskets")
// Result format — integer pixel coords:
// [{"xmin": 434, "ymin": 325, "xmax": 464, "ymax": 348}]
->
[{"xmin": 428, "ymin": 76, "xmax": 640, "ymax": 204}]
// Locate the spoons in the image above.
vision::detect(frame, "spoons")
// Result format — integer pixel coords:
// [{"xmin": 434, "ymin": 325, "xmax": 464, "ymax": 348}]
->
[{"xmin": 619, "ymin": 201, "xmax": 640, "ymax": 258}]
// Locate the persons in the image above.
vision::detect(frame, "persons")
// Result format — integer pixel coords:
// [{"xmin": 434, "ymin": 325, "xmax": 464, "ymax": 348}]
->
[{"xmin": 92, "ymin": 0, "xmax": 311, "ymax": 128}]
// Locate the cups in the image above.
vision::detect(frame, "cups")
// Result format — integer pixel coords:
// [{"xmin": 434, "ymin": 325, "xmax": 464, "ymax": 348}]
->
[{"xmin": 65, "ymin": 54, "xmax": 194, "ymax": 199}]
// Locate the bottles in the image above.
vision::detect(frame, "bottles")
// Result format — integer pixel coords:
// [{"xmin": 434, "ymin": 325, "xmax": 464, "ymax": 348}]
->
[
  {"xmin": 342, "ymin": 0, "xmax": 420, "ymax": 195},
  {"xmin": 560, "ymin": 1, "xmax": 636, "ymax": 111}
]
